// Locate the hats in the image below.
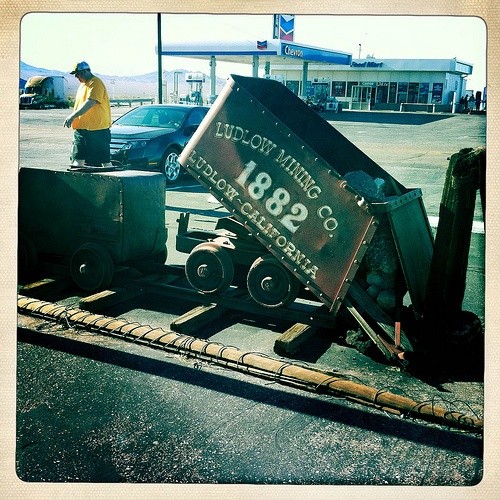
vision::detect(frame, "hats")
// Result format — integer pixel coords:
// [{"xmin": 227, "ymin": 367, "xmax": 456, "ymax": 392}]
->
[{"xmin": 70, "ymin": 62, "xmax": 90, "ymax": 75}]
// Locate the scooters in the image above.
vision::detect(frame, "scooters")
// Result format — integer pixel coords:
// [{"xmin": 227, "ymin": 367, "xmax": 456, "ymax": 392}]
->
[{"xmin": 305, "ymin": 97, "xmax": 325, "ymax": 114}]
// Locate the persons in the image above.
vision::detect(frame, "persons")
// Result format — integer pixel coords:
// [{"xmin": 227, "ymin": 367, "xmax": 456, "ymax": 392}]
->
[
  {"xmin": 64, "ymin": 61, "xmax": 113, "ymax": 168},
  {"xmin": 459, "ymin": 94, "xmax": 480, "ymax": 115}
]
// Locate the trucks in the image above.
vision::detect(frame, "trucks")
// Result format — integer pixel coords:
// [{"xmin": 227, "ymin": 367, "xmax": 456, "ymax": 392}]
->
[{"xmin": 19, "ymin": 75, "xmax": 66, "ymax": 110}]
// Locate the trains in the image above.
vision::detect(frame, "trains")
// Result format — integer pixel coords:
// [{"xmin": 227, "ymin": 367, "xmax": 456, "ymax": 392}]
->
[{"xmin": 17, "ymin": 74, "xmax": 486, "ymax": 364}]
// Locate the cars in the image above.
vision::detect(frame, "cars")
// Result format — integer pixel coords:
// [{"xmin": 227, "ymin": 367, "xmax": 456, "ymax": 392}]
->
[{"xmin": 66, "ymin": 104, "xmax": 213, "ymax": 185}]
